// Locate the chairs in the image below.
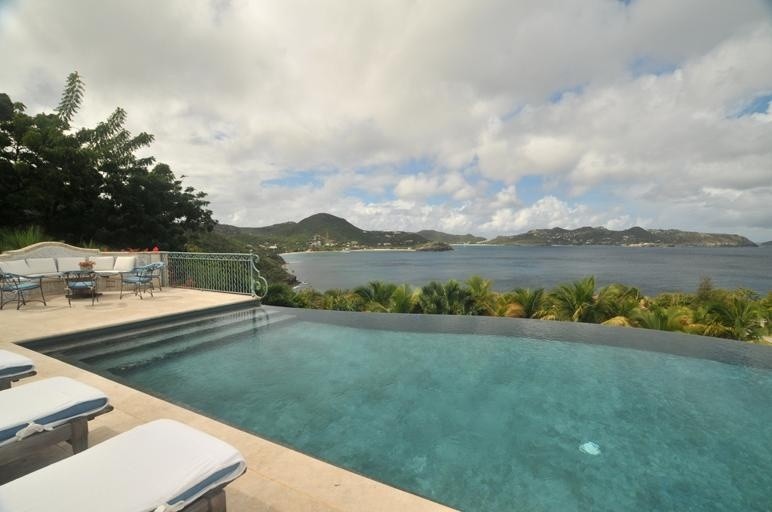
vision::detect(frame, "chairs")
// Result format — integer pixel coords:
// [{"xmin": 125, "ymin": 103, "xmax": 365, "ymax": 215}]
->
[
  {"xmin": 0, "ymin": 348, "xmax": 37, "ymax": 388},
  {"xmin": 1, "ymin": 416, "xmax": 247, "ymax": 512},
  {"xmin": 2, "ymin": 376, "xmax": 114, "ymax": 465},
  {"xmin": 1, "ymin": 256, "xmax": 165, "ymax": 311}
]
